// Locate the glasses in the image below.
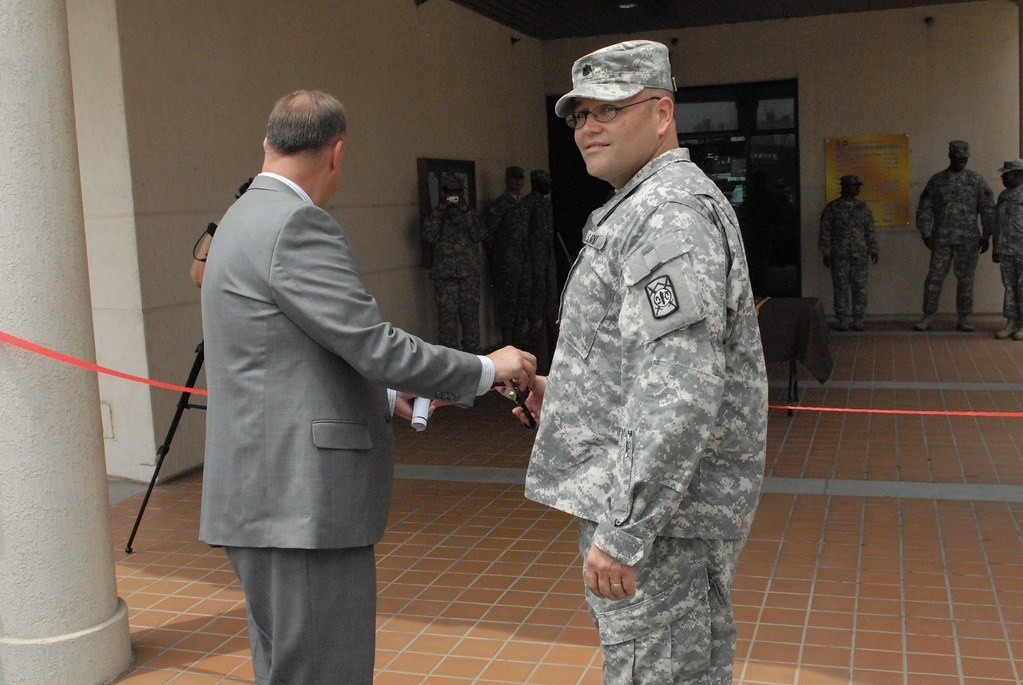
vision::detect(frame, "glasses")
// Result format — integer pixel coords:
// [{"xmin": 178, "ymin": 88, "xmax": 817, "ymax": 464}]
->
[{"xmin": 566, "ymin": 96, "xmax": 662, "ymax": 129}]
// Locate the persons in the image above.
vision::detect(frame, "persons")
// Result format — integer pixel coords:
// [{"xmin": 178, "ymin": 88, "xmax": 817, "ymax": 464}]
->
[
  {"xmin": 422, "ymin": 176, "xmax": 488, "ymax": 356},
  {"xmin": 992, "ymin": 162, "xmax": 1023, "ymax": 339},
  {"xmin": 198, "ymin": 90, "xmax": 535, "ymax": 683},
  {"xmin": 521, "ymin": 170, "xmax": 558, "ymax": 360},
  {"xmin": 917, "ymin": 141, "xmax": 995, "ymax": 329},
  {"xmin": 513, "ymin": 38, "xmax": 767, "ymax": 685},
  {"xmin": 487, "ymin": 167, "xmax": 531, "ymax": 346},
  {"xmin": 819, "ymin": 177, "xmax": 879, "ymax": 330}
]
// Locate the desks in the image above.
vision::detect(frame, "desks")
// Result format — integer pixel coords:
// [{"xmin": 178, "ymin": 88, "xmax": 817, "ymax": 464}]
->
[{"xmin": 753, "ymin": 297, "xmax": 832, "ymax": 418}]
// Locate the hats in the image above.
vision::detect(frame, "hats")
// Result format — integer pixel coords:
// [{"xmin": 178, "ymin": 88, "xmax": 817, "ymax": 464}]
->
[
  {"xmin": 530, "ymin": 170, "xmax": 552, "ymax": 182},
  {"xmin": 998, "ymin": 158, "xmax": 1023, "ymax": 173},
  {"xmin": 840, "ymin": 174, "xmax": 863, "ymax": 186},
  {"xmin": 554, "ymin": 40, "xmax": 678, "ymax": 120},
  {"xmin": 506, "ymin": 166, "xmax": 525, "ymax": 178},
  {"xmin": 949, "ymin": 140, "xmax": 971, "ymax": 158},
  {"xmin": 441, "ymin": 173, "xmax": 467, "ymax": 189}
]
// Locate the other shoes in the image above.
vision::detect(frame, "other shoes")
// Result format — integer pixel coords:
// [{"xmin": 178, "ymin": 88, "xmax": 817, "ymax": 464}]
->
[
  {"xmin": 915, "ymin": 313, "xmax": 935, "ymax": 330},
  {"xmin": 996, "ymin": 319, "xmax": 1023, "ymax": 339},
  {"xmin": 840, "ymin": 317, "xmax": 864, "ymax": 330},
  {"xmin": 957, "ymin": 314, "xmax": 977, "ymax": 332}
]
